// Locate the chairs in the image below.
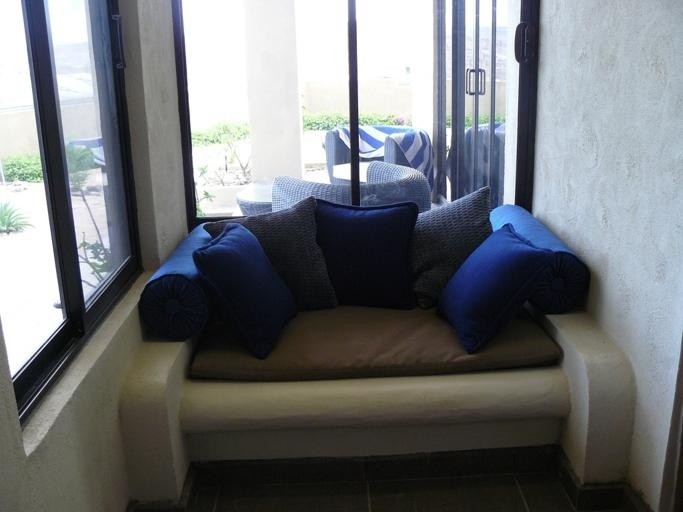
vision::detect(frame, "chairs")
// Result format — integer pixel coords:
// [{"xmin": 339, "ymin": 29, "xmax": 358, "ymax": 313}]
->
[
  {"xmin": 325, "ymin": 126, "xmax": 433, "ymax": 193},
  {"xmin": 271, "ymin": 161, "xmax": 431, "ymax": 212},
  {"xmin": 443, "ymin": 122, "xmax": 503, "ymax": 212}
]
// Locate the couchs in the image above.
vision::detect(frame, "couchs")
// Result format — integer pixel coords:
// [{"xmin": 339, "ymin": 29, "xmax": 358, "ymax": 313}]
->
[{"xmin": 118, "ymin": 203, "xmax": 638, "ymax": 503}]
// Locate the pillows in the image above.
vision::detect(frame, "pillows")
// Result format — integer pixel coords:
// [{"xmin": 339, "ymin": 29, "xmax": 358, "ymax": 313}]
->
[
  {"xmin": 192, "ymin": 223, "xmax": 291, "ymax": 359},
  {"xmin": 412, "ymin": 186, "xmax": 493, "ymax": 307},
  {"xmin": 436, "ymin": 222, "xmax": 549, "ymax": 353},
  {"xmin": 203, "ymin": 195, "xmax": 338, "ymax": 311},
  {"xmin": 314, "ymin": 198, "xmax": 418, "ymax": 311}
]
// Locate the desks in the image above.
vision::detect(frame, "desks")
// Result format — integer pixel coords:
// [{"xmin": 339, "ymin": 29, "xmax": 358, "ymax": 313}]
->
[{"xmin": 237, "ymin": 183, "xmax": 272, "ymax": 216}]
